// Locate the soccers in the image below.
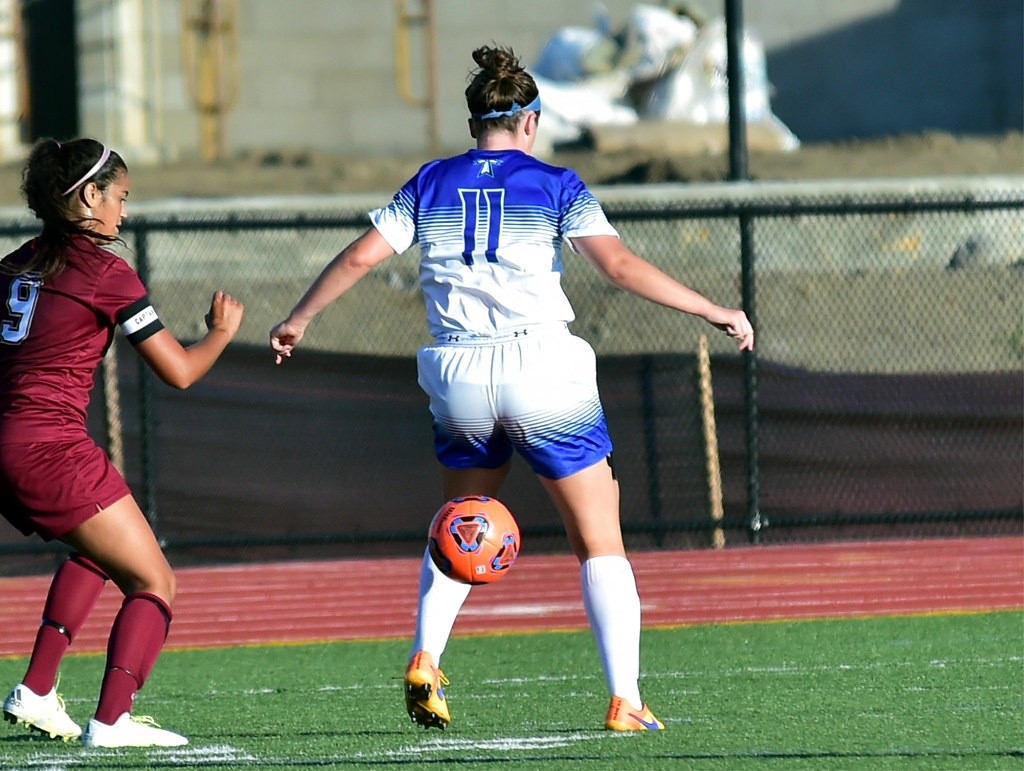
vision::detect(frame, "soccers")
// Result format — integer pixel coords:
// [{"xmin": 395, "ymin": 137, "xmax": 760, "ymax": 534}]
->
[{"xmin": 426, "ymin": 493, "xmax": 522, "ymax": 587}]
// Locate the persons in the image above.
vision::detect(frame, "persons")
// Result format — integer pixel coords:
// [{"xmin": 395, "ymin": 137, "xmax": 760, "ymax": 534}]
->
[
  {"xmin": 0, "ymin": 136, "xmax": 246, "ymax": 748},
  {"xmin": 269, "ymin": 39, "xmax": 756, "ymax": 731}
]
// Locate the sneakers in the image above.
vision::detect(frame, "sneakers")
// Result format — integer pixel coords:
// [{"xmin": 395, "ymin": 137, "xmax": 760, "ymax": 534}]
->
[
  {"xmin": 605, "ymin": 695, "xmax": 667, "ymax": 732},
  {"xmin": 404, "ymin": 653, "xmax": 452, "ymax": 730},
  {"xmin": 3, "ymin": 684, "xmax": 82, "ymax": 742},
  {"xmin": 84, "ymin": 713, "xmax": 188, "ymax": 749}
]
